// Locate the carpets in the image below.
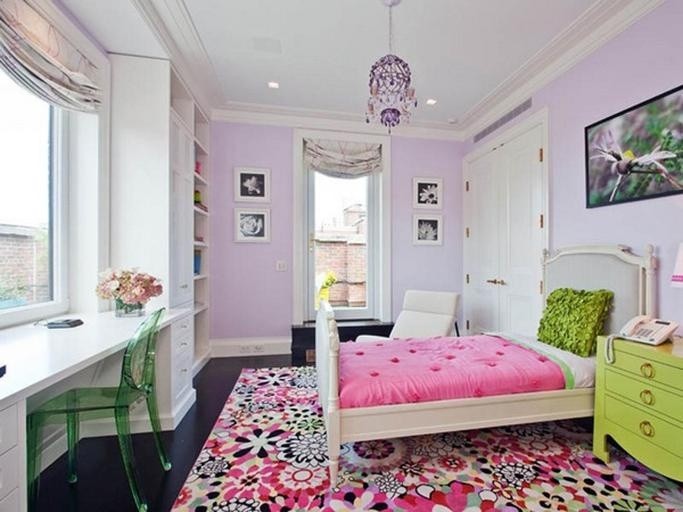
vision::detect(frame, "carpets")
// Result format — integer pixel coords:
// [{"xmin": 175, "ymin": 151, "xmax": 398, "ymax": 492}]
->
[{"xmin": 168, "ymin": 368, "xmax": 682, "ymax": 512}]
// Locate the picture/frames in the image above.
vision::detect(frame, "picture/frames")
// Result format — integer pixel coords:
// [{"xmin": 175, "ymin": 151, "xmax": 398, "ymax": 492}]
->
[
  {"xmin": 584, "ymin": 85, "xmax": 683, "ymax": 209},
  {"xmin": 412, "ymin": 177, "xmax": 445, "ymax": 210},
  {"xmin": 233, "ymin": 166, "xmax": 271, "ymax": 203},
  {"xmin": 234, "ymin": 207, "xmax": 271, "ymax": 243},
  {"xmin": 412, "ymin": 213, "xmax": 443, "ymax": 245}
]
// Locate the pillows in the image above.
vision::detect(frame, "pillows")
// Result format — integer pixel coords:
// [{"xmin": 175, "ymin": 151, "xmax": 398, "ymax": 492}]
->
[{"xmin": 537, "ymin": 288, "xmax": 614, "ymax": 358}]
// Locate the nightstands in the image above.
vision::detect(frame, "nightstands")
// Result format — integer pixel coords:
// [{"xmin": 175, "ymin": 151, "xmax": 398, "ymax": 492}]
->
[{"xmin": 593, "ymin": 334, "xmax": 683, "ymax": 483}]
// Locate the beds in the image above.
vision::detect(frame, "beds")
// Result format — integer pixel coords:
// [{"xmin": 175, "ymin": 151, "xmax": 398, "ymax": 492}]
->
[{"xmin": 315, "ymin": 244, "xmax": 658, "ymax": 490}]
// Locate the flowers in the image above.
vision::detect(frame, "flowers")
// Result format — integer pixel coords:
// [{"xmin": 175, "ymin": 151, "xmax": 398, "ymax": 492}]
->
[
  {"xmin": 95, "ymin": 270, "xmax": 163, "ymax": 305},
  {"xmin": 322, "ymin": 270, "xmax": 336, "ymax": 288}
]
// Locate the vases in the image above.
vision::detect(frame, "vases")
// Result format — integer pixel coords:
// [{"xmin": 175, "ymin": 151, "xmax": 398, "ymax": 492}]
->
[
  {"xmin": 319, "ymin": 288, "xmax": 329, "ymax": 300},
  {"xmin": 114, "ymin": 298, "xmax": 142, "ymax": 317}
]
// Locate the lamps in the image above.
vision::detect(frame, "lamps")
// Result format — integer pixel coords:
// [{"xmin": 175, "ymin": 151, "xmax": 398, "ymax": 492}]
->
[{"xmin": 365, "ymin": 1, "xmax": 417, "ymax": 135}]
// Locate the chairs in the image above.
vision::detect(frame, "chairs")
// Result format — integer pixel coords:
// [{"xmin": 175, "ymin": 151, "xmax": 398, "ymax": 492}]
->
[
  {"xmin": 356, "ymin": 290, "xmax": 462, "ymax": 343},
  {"xmin": 27, "ymin": 307, "xmax": 172, "ymax": 512}
]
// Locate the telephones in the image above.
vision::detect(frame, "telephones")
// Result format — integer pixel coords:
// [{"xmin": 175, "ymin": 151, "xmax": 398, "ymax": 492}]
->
[{"xmin": 620, "ymin": 315, "xmax": 679, "ymax": 346}]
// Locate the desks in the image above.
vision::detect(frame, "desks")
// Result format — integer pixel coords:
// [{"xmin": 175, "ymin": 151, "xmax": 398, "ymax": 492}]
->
[{"xmin": 291, "ymin": 321, "xmax": 394, "ymax": 366}]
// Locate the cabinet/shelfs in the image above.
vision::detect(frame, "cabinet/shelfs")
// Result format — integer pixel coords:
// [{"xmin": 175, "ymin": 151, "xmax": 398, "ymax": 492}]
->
[
  {"xmin": 80, "ymin": 58, "xmax": 212, "ymax": 380},
  {"xmin": 0, "ymin": 308, "xmax": 197, "ymax": 512}
]
